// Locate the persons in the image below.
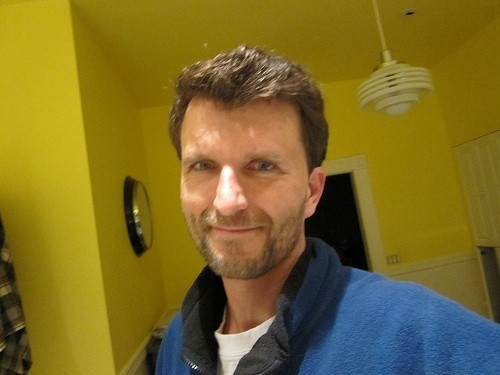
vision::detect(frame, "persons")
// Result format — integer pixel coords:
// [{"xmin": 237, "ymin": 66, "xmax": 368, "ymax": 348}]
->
[{"xmin": 152, "ymin": 44, "xmax": 500, "ymax": 375}]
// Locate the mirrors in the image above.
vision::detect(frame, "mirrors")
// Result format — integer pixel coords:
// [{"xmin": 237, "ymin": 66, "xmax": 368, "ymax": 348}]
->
[{"xmin": 124, "ymin": 176, "xmax": 154, "ymax": 257}]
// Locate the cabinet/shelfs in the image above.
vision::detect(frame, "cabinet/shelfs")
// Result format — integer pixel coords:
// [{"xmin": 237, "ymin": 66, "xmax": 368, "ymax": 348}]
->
[{"xmin": 450, "ymin": 129, "xmax": 500, "ymax": 324}]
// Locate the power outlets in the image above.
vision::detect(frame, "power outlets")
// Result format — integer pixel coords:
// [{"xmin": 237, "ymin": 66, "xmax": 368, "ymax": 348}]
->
[{"xmin": 386, "ymin": 253, "xmax": 401, "ymax": 264}]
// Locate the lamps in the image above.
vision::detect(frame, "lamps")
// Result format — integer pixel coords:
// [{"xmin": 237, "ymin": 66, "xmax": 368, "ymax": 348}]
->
[{"xmin": 354, "ymin": 0, "xmax": 436, "ymax": 118}]
